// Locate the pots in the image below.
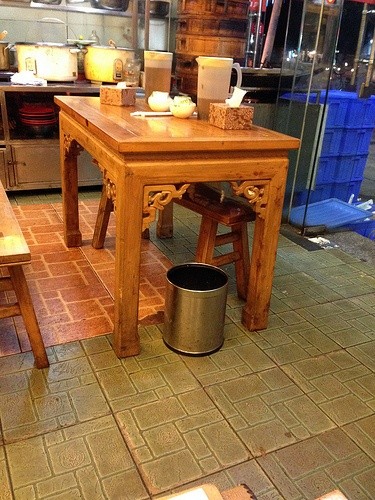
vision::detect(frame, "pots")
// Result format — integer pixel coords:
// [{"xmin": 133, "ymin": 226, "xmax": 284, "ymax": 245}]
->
[
  {"xmin": 231, "ymin": 65, "xmax": 325, "ymax": 101},
  {"xmin": 32, "ymin": 0, "xmax": 170, "ymax": 16},
  {"xmin": 0, "ymin": 42, "xmax": 10, "ymax": 70}
]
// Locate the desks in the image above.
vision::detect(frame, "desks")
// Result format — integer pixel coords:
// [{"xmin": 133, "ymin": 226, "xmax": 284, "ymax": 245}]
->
[{"xmin": 52, "ymin": 94, "xmax": 301, "ymax": 358}]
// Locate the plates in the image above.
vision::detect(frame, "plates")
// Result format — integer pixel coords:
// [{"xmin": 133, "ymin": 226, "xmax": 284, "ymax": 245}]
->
[{"xmin": 67, "ymin": 39, "xmax": 96, "ymax": 45}]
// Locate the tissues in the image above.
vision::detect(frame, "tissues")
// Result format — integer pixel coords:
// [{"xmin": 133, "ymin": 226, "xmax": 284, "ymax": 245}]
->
[
  {"xmin": 208, "ymin": 85, "xmax": 256, "ymax": 131},
  {"xmin": 99, "ymin": 81, "xmax": 136, "ymax": 108}
]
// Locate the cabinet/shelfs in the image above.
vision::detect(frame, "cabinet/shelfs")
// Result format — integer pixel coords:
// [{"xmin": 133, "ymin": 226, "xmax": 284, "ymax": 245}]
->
[{"xmin": 0, "ymin": 79, "xmax": 145, "ymax": 191}]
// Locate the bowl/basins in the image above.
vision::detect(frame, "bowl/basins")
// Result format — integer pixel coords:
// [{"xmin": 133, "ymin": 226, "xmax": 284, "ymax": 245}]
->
[
  {"xmin": 18, "ymin": 107, "xmax": 57, "ymax": 135},
  {"xmin": 170, "ymin": 100, "xmax": 196, "ymax": 119},
  {"xmin": 148, "ymin": 97, "xmax": 173, "ymax": 112}
]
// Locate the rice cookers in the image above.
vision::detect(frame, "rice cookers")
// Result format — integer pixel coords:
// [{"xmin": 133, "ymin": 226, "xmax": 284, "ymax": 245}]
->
[
  {"xmin": 82, "ymin": 44, "xmax": 135, "ymax": 84},
  {"xmin": 13, "ymin": 41, "xmax": 81, "ymax": 83}
]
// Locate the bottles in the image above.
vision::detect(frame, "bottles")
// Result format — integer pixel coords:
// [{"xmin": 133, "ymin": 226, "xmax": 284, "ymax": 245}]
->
[
  {"xmin": 88, "ymin": 30, "xmax": 99, "ymax": 45},
  {"xmin": 124, "ymin": 58, "xmax": 141, "ymax": 87}
]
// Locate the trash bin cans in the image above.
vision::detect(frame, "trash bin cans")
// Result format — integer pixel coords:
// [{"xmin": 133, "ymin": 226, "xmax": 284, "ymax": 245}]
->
[{"xmin": 163, "ymin": 262, "xmax": 229, "ymax": 357}]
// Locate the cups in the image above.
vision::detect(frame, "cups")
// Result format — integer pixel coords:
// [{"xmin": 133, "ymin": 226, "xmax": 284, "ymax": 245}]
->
[{"xmin": 144, "ymin": 50, "xmax": 173, "ymax": 103}]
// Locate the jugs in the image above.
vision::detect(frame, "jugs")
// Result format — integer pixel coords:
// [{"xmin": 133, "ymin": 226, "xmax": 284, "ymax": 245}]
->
[{"xmin": 196, "ymin": 56, "xmax": 242, "ymax": 120}]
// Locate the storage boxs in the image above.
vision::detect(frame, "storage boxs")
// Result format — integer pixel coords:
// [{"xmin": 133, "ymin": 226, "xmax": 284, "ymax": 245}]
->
[{"xmin": 283, "ymin": 88, "xmax": 375, "ymax": 206}]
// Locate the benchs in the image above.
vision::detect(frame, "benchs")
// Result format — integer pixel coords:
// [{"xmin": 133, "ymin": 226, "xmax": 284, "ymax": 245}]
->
[
  {"xmin": 0, "ymin": 179, "xmax": 50, "ymax": 370},
  {"xmin": 91, "ymin": 158, "xmax": 257, "ymax": 302}
]
